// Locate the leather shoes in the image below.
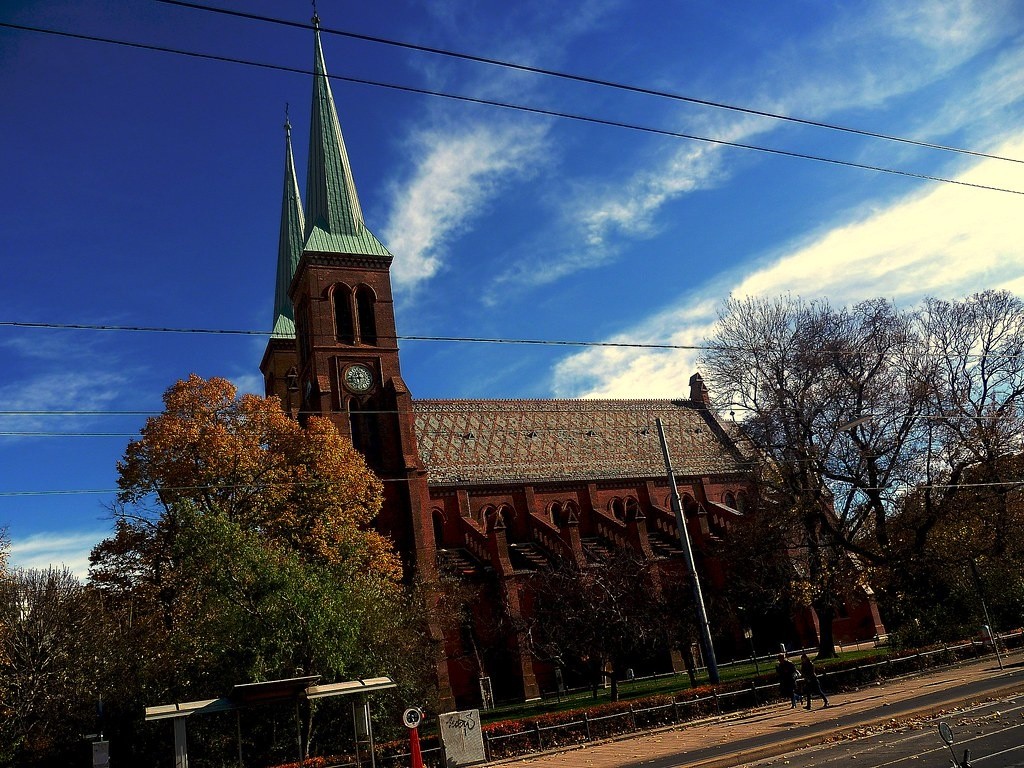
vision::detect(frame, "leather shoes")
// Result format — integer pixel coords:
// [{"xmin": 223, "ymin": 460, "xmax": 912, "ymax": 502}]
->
[
  {"xmin": 823, "ymin": 701, "xmax": 830, "ymax": 707},
  {"xmin": 803, "ymin": 705, "xmax": 812, "ymax": 710},
  {"xmin": 800, "ymin": 696, "xmax": 804, "ymax": 705},
  {"xmin": 790, "ymin": 704, "xmax": 796, "ymax": 709}
]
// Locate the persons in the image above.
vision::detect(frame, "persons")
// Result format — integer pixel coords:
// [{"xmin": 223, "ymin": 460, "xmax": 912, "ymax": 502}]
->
[
  {"xmin": 775, "ymin": 652, "xmax": 803, "ymax": 709},
  {"xmin": 801, "ymin": 653, "xmax": 829, "ymax": 710}
]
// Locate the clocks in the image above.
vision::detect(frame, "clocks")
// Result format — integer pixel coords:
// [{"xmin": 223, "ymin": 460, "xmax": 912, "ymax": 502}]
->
[{"xmin": 341, "ymin": 361, "xmax": 379, "ymax": 394}]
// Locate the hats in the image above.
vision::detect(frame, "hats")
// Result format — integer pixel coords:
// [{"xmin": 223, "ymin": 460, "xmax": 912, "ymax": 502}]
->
[{"xmin": 777, "ymin": 653, "xmax": 787, "ymax": 661}]
